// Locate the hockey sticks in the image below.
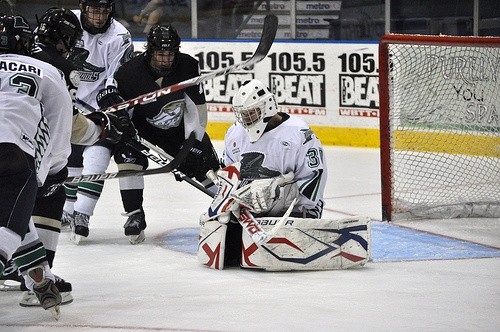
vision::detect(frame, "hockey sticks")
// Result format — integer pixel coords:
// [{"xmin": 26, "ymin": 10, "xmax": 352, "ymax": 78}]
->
[
  {"xmin": 206, "ymin": 169, "xmax": 298, "ymax": 246},
  {"xmin": 63, "ymin": 130, "xmax": 196, "ymax": 183},
  {"xmin": 75, "ymin": 97, "xmax": 217, "ymax": 199},
  {"xmin": 96, "ymin": 14, "xmax": 278, "ymax": 113}
]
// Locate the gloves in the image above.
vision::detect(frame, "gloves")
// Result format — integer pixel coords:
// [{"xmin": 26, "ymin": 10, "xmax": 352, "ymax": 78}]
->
[
  {"xmin": 173, "ymin": 139, "xmax": 206, "ymax": 181},
  {"xmin": 86, "ymin": 87, "xmax": 137, "ymax": 142}
]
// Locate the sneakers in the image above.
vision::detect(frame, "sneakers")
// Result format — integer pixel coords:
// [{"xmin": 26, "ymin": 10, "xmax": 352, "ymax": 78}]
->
[
  {"xmin": 60, "ymin": 211, "xmax": 75, "ymax": 230},
  {"xmin": 33, "ymin": 278, "xmax": 63, "ymax": 321},
  {"xmin": 17, "ymin": 275, "xmax": 74, "ymax": 307},
  {"xmin": 121, "ymin": 207, "xmax": 148, "ymax": 245},
  {"xmin": 70, "ymin": 210, "xmax": 91, "ymax": 245}
]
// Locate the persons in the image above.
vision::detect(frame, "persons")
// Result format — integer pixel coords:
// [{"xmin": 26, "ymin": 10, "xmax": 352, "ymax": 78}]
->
[
  {"xmin": 0, "ymin": 0, "xmax": 222, "ymax": 320},
  {"xmin": 198, "ymin": 78, "xmax": 371, "ymax": 271}
]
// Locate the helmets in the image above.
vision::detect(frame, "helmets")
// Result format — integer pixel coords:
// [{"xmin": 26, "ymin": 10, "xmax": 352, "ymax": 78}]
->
[
  {"xmin": 35, "ymin": 7, "xmax": 83, "ymax": 59},
  {"xmin": 79, "ymin": 0, "xmax": 115, "ymax": 35},
  {"xmin": 231, "ymin": 79, "xmax": 279, "ymax": 143},
  {"xmin": 0, "ymin": 15, "xmax": 36, "ymax": 59},
  {"xmin": 146, "ymin": 24, "xmax": 181, "ymax": 76}
]
